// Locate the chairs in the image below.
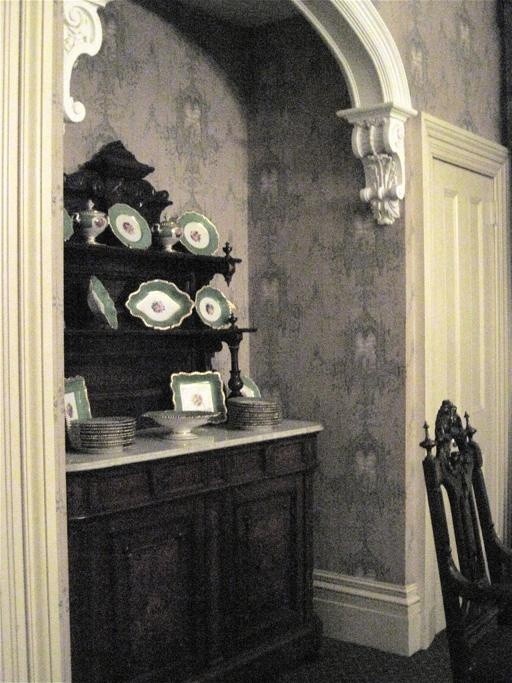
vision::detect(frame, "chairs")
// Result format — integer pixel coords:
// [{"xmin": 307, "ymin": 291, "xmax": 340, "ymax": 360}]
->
[{"xmin": 419, "ymin": 398, "xmax": 511, "ymax": 682}]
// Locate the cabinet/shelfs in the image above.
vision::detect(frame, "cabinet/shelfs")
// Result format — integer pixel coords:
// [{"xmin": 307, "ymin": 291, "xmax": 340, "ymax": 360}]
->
[
  {"xmin": 64, "ymin": 141, "xmax": 256, "ymax": 418},
  {"xmin": 67, "ymin": 435, "xmax": 324, "ymax": 680}
]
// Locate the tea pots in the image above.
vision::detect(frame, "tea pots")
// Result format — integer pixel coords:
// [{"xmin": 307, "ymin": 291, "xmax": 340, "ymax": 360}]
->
[
  {"xmin": 69, "ymin": 198, "xmax": 109, "ymax": 247},
  {"xmin": 153, "ymin": 210, "xmax": 184, "ymax": 253}
]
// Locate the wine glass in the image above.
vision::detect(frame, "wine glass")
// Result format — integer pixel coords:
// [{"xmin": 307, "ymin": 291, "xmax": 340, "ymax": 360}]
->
[{"xmin": 141, "ymin": 409, "xmax": 220, "ymax": 442}]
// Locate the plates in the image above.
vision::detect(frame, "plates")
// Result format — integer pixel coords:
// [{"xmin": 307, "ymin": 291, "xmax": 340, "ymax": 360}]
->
[
  {"xmin": 64, "ymin": 374, "xmax": 93, "ymax": 436},
  {"xmin": 169, "ymin": 370, "xmax": 229, "ymax": 425},
  {"xmin": 107, "ymin": 202, "xmax": 153, "ymax": 250},
  {"xmin": 225, "ymin": 375, "xmax": 262, "ymax": 398},
  {"xmin": 124, "ymin": 278, "xmax": 195, "ymax": 331},
  {"xmin": 66, "ymin": 415, "xmax": 138, "ymax": 456},
  {"xmin": 195, "ymin": 284, "xmax": 231, "ymax": 329},
  {"xmin": 225, "ymin": 395, "xmax": 283, "ymax": 429},
  {"xmin": 86, "ymin": 275, "xmax": 119, "ymax": 330},
  {"xmin": 176, "ymin": 210, "xmax": 220, "ymax": 256}
]
